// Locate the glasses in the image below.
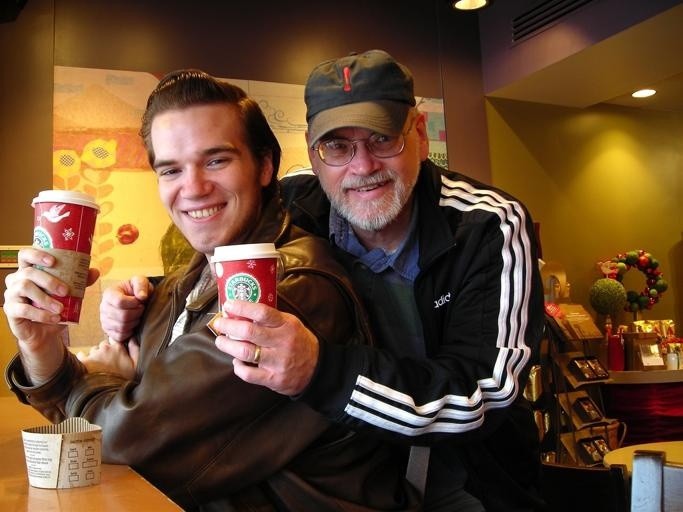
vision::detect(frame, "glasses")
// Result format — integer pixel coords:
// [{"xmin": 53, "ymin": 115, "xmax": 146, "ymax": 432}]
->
[{"xmin": 309, "ymin": 121, "xmax": 414, "ymax": 167}]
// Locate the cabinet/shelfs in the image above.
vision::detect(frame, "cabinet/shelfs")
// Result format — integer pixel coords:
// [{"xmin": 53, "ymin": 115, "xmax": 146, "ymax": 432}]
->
[{"xmin": 540, "ymin": 300, "xmax": 626, "ymax": 472}]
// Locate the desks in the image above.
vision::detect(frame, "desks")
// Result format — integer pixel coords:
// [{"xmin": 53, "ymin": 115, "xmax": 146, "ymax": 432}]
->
[{"xmin": 1, "ymin": 305, "xmax": 183, "ymax": 512}]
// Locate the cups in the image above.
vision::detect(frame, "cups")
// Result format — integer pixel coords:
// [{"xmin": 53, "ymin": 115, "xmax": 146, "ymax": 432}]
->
[
  {"xmin": 210, "ymin": 242, "xmax": 281, "ymax": 346},
  {"xmin": 30, "ymin": 190, "xmax": 101, "ymax": 326}
]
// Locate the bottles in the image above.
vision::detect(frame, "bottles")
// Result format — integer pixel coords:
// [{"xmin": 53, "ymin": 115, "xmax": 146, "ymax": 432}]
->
[
  {"xmin": 607, "ymin": 328, "xmax": 625, "ymax": 372},
  {"xmin": 666, "ymin": 343, "xmax": 683, "ymax": 371},
  {"xmin": 549, "ymin": 278, "xmax": 571, "ymax": 300}
]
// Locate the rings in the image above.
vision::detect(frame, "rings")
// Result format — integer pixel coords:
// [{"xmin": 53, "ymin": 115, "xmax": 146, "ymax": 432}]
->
[{"xmin": 254, "ymin": 346, "xmax": 260, "ymax": 363}]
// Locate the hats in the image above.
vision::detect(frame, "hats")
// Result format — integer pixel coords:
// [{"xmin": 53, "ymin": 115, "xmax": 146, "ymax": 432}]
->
[{"xmin": 305, "ymin": 51, "xmax": 415, "ymax": 149}]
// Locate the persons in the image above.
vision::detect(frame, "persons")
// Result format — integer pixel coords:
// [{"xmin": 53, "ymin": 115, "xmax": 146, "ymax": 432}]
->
[
  {"xmin": 212, "ymin": 47, "xmax": 539, "ymax": 512},
  {"xmin": 1, "ymin": 67, "xmax": 375, "ymax": 512}
]
